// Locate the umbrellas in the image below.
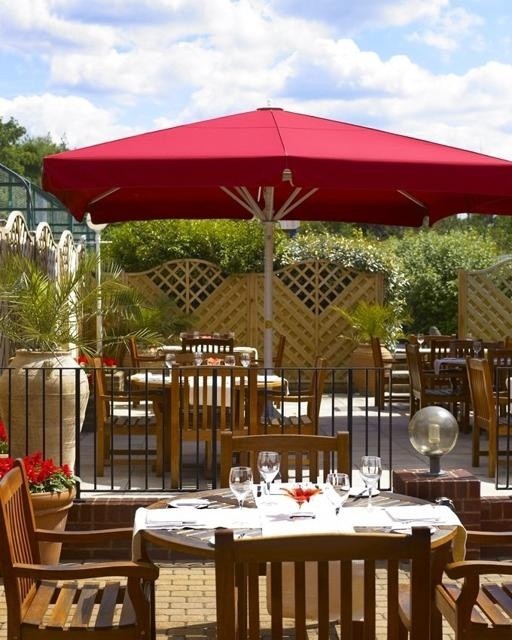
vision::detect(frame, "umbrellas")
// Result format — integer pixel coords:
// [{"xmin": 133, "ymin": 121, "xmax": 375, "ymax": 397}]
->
[{"xmin": 42, "ymin": 109, "xmax": 511, "ymax": 378}]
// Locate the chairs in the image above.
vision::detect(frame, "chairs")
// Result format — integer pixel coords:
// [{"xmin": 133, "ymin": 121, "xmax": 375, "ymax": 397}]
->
[{"xmin": 0, "ymin": 456, "xmax": 159, "ymax": 639}]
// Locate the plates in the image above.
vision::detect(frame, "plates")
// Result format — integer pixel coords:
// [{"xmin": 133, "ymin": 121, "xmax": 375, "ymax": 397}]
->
[
  {"xmin": 168, "ymin": 498, "xmax": 209, "ymax": 510},
  {"xmin": 347, "ymin": 486, "xmax": 381, "ymax": 498},
  {"xmin": 390, "ymin": 521, "xmax": 438, "ymax": 537},
  {"xmin": 206, "ymin": 534, "xmax": 251, "ymax": 546}
]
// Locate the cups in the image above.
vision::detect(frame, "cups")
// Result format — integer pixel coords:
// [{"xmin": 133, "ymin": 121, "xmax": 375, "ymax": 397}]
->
[
  {"xmin": 240, "ymin": 352, "xmax": 251, "ymax": 367},
  {"xmin": 223, "ymin": 356, "xmax": 235, "ymax": 366},
  {"xmin": 193, "ymin": 351, "xmax": 204, "ymax": 366}
]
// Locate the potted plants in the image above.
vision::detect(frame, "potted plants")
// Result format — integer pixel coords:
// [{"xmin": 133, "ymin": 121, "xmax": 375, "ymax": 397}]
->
[
  {"xmin": 328, "ymin": 297, "xmax": 409, "ymax": 398},
  {"xmin": 0, "ymin": 249, "xmax": 168, "ymax": 475}
]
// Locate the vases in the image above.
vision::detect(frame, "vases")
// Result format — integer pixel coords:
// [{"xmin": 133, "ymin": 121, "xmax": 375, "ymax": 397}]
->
[{"xmin": 30, "ymin": 485, "xmax": 77, "ymax": 566}]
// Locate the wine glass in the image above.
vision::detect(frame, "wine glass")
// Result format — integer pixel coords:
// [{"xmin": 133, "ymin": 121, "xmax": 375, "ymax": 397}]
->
[
  {"xmin": 471, "ymin": 340, "xmax": 483, "ymax": 358},
  {"xmin": 360, "ymin": 452, "xmax": 383, "ymax": 511},
  {"xmin": 323, "ymin": 473, "xmax": 352, "ymax": 530},
  {"xmin": 417, "ymin": 333, "xmax": 425, "ymax": 352},
  {"xmin": 164, "ymin": 353, "xmax": 177, "ymax": 382},
  {"xmin": 227, "ymin": 464, "xmax": 255, "ymax": 520},
  {"xmin": 257, "ymin": 450, "xmax": 280, "ymax": 503}
]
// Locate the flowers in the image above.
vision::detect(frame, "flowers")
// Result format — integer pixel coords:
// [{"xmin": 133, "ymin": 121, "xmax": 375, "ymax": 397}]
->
[{"xmin": 0, "ymin": 420, "xmax": 84, "ymax": 495}]
